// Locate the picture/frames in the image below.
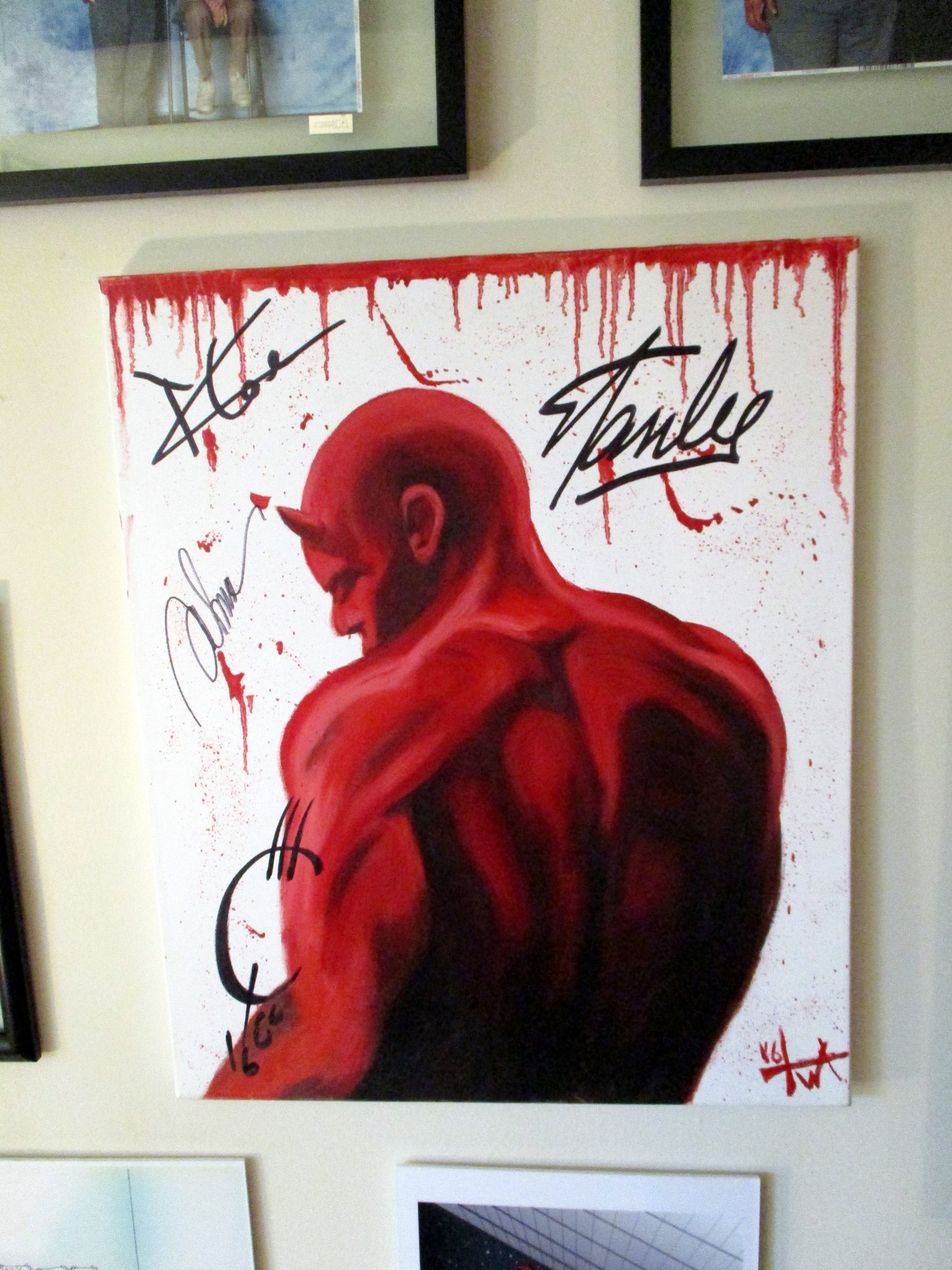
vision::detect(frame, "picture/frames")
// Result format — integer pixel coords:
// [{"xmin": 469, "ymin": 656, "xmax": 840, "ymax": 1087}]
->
[
  {"xmin": 0, "ymin": 0, "xmax": 467, "ymax": 206},
  {"xmin": 639, "ymin": 0, "xmax": 952, "ymax": 182}
]
